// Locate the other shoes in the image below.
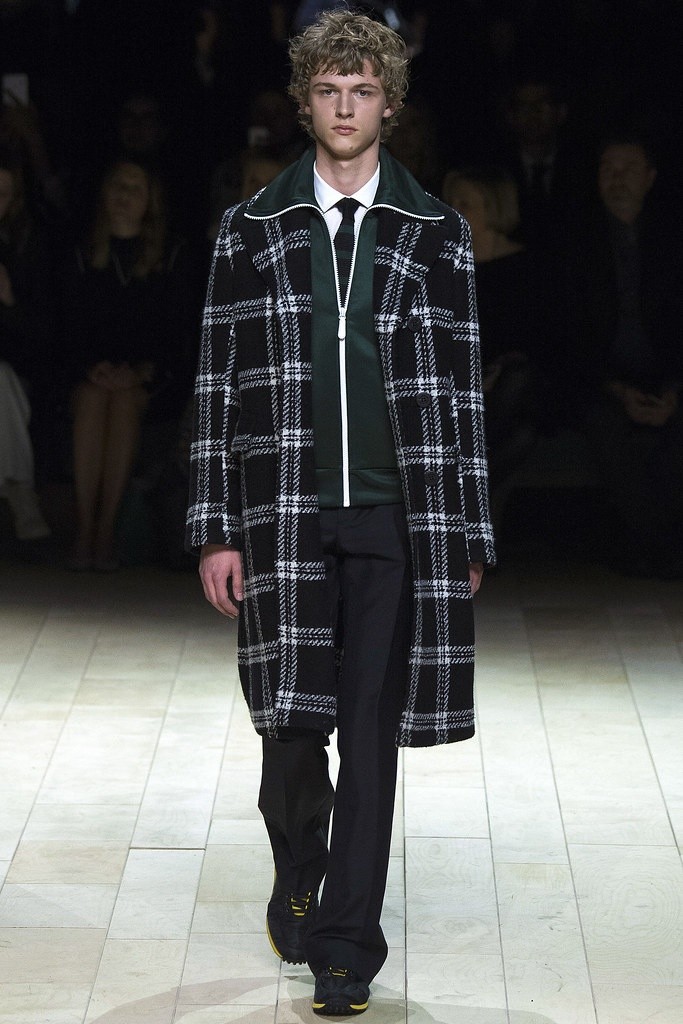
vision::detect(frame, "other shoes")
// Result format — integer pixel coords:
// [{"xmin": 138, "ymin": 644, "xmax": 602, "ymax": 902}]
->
[{"xmin": 9, "ymin": 491, "xmax": 49, "ymax": 540}]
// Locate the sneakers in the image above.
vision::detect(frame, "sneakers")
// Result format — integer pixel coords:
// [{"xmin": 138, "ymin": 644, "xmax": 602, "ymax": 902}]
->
[
  {"xmin": 312, "ymin": 968, "xmax": 370, "ymax": 1014},
  {"xmin": 266, "ymin": 870, "xmax": 319, "ymax": 966}
]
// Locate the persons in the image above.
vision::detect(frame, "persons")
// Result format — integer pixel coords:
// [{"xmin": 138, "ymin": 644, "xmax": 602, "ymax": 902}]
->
[
  {"xmin": 0, "ymin": 1, "xmax": 683, "ymax": 571},
  {"xmin": 185, "ymin": 9, "xmax": 498, "ymax": 1016}
]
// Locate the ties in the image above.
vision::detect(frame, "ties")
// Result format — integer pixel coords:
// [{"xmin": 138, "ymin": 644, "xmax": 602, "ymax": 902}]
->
[{"xmin": 333, "ymin": 198, "xmax": 360, "ymax": 307}]
